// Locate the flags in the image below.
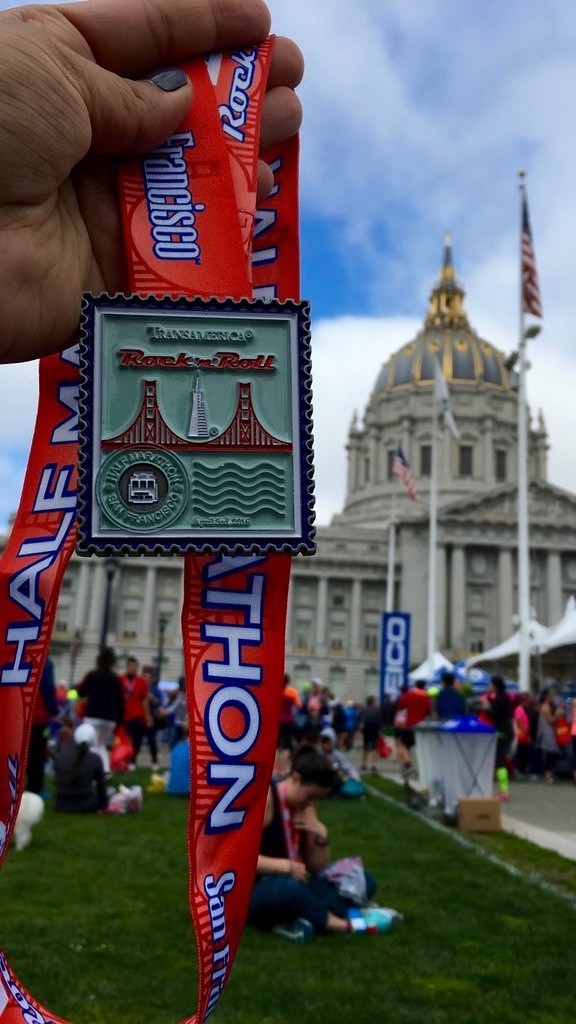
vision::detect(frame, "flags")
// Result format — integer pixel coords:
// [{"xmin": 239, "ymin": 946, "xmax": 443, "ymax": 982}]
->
[
  {"xmin": 518, "ymin": 188, "xmax": 543, "ymax": 319},
  {"xmin": 391, "ymin": 446, "xmax": 421, "ymax": 501},
  {"xmin": 434, "ymin": 358, "xmax": 459, "ymax": 442}
]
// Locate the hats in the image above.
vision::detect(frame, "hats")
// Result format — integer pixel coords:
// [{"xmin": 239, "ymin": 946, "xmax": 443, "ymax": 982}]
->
[{"xmin": 73, "ymin": 724, "xmax": 97, "ymax": 747}]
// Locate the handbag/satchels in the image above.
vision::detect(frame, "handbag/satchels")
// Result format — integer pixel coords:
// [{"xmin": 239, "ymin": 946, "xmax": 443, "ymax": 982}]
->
[{"xmin": 326, "ymin": 855, "xmax": 366, "ymax": 906}]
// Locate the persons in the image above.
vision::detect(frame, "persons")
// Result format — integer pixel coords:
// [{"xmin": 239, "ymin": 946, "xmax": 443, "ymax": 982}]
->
[
  {"xmin": 22, "ymin": 646, "xmax": 575, "ymax": 942},
  {"xmin": 0, "ymin": 0, "xmax": 303, "ymax": 364}
]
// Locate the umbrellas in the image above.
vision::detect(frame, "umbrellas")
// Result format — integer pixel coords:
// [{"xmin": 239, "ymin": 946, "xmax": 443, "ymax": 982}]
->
[{"xmin": 406, "ymin": 596, "xmax": 576, "ymax": 702}]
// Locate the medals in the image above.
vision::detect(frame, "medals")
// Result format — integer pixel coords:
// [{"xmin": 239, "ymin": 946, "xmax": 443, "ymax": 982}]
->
[{"xmin": 78, "ymin": 289, "xmax": 318, "ymax": 559}]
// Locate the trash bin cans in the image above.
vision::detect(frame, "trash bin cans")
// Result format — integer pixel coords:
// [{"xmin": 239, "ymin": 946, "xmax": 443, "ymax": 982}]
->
[
  {"xmin": 412, "ymin": 715, "xmax": 443, "ymax": 809},
  {"xmin": 435, "ymin": 715, "xmax": 500, "ymax": 828}
]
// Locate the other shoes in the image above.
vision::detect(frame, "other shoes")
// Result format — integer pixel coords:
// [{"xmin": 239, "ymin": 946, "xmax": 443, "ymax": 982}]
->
[
  {"xmin": 346, "ymin": 909, "xmax": 393, "ymax": 936},
  {"xmin": 274, "ymin": 920, "xmax": 315, "ymax": 942}
]
